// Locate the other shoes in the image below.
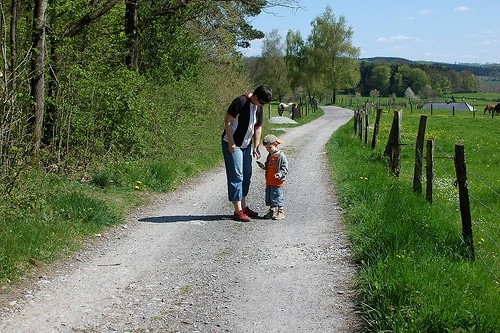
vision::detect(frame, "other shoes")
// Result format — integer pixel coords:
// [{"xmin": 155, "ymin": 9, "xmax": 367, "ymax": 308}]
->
[
  {"xmin": 264, "ymin": 210, "xmax": 275, "ymax": 218},
  {"xmin": 272, "ymin": 210, "xmax": 285, "ymax": 219}
]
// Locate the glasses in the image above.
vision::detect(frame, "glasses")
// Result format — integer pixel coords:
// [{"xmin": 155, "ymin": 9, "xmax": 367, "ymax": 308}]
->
[{"xmin": 259, "ymin": 100, "xmax": 266, "ymax": 105}]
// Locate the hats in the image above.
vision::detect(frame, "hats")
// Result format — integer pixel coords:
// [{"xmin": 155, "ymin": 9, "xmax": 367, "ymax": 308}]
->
[{"xmin": 263, "ymin": 134, "xmax": 282, "ymax": 145}]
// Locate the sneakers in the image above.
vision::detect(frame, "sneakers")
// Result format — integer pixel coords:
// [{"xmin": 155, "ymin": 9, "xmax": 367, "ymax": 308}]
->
[
  {"xmin": 234, "ymin": 210, "xmax": 251, "ymax": 221},
  {"xmin": 243, "ymin": 207, "xmax": 258, "ymax": 217}
]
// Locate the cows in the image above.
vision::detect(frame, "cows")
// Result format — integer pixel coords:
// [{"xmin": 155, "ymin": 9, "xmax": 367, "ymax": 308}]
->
[
  {"xmin": 278, "ymin": 103, "xmax": 298, "ymax": 116},
  {"xmin": 484, "ymin": 103, "xmax": 500, "ymax": 116}
]
